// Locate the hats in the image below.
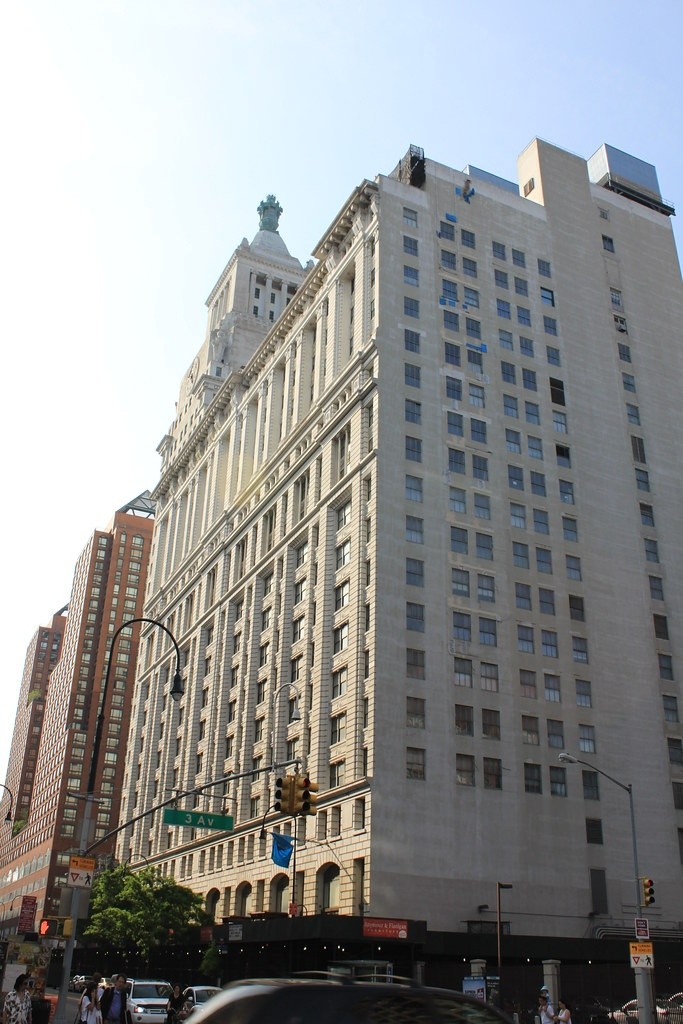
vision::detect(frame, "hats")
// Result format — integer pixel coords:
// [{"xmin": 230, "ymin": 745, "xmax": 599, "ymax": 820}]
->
[{"xmin": 541, "ymin": 986, "xmax": 549, "ymax": 991}]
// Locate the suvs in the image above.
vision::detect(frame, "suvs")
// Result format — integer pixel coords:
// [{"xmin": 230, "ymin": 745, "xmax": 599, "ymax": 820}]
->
[{"xmin": 123, "ymin": 977, "xmax": 176, "ymax": 1024}]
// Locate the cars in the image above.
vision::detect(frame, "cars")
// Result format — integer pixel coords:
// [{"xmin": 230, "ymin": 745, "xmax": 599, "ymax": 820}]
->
[
  {"xmin": 667, "ymin": 992, "xmax": 683, "ymax": 1008},
  {"xmin": 183, "ymin": 970, "xmax": 515, "ymax": 1024},
  {"xmin": 181, "ymin": 986, "xmax": 224, "ymax": 1016},
  {"xmin": 607, "ymin": 997, "xmax": 683, "ymax": 1024},
  {"xmin": 69, "ymin": 973, "xmax": 117, "ymax": 994}
]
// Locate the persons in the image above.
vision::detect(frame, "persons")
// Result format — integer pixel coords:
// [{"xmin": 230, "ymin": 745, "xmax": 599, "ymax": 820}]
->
[
  {"xmin": 2, "ymin": 974, "xmax": 32, "ymax": 1024},
  {"xmin": 538, "ymin": 985, "xmax": 554, "ymax": 1024},
  {"xmin": 77, "ymin": 972, "xmax": 128, "ymax": 1024},
  {"xmin": 167, "ymin": 985, "xmax": 189, "ymax": 1012},
  {"xmin": 553, "ymin": 999, "xmax": 571, "ymax": 1024}
]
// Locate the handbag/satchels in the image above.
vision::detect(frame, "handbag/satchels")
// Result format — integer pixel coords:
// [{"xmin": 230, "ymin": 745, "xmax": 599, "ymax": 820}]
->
[{"xmin": 77, "ymin": 1019, "xmax": 86, "ymax": 1024}]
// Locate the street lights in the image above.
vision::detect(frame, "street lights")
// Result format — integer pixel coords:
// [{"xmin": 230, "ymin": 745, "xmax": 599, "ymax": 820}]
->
[
  {"xmin": 556, "ymin": 752, "xmax": 659, "ymax": 1024},
  {"xmin": 48, "ymin": 617, "xmax": 184, "ymax": 1024},
  {"xmin": 496, "ymin": 882, "xmax": 514, "ymax": 967}
]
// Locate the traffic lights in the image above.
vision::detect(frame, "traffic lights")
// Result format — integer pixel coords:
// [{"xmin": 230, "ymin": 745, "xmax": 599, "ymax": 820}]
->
[
  {"xmin": 307, "ymin": 782, "xmax": 320, "ymax": 815},
  {"xmin": 39, "ymin": 918, "xmax": 59, "ymax": 936},
  {"xmin": 293, "ymin": 776, "xmax": 311, "ymax": 815},
  {"xmin": 272, "ymin": 775, "xmax": 293, "ymax": 815},
  {"xmin": 643, "ymin": 878, "xmax": 656, "ymax": 906}
]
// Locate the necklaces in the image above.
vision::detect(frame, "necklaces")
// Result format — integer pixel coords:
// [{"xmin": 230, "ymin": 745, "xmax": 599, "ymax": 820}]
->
[{"xmin": 559, "ymin": 1010, "xmax": 564, "ymax": 1016}]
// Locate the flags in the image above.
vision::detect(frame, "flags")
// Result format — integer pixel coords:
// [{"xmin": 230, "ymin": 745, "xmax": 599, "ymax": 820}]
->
[{"xmin": 270, "ymin": 833, "xmax": 301, "ymax": 868}]
